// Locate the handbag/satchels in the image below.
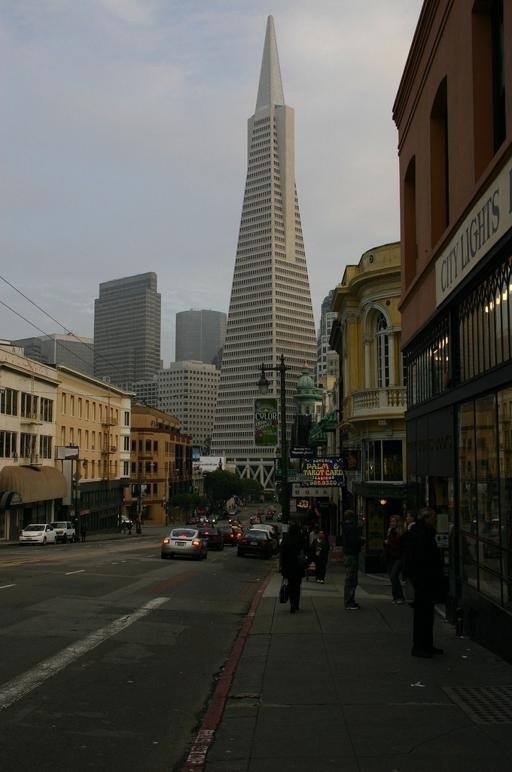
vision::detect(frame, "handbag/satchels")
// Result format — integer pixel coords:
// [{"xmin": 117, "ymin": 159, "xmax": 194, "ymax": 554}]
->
[{"xmin": 279, "ymin": 584, "xmax": 288, "ymax": 602}]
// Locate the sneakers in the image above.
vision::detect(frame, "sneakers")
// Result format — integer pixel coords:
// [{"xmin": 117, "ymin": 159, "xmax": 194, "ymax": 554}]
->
[
  {"xmin": 392, "ymin": 599, "xmax": 404, "ymax": 604},
  {"xmin": 317, "ymin": 579, "xmax": 324, "ymax": 584},
  {"xmin": 344, "ymin": 603, "xmax": 359, "ymax": 610}
]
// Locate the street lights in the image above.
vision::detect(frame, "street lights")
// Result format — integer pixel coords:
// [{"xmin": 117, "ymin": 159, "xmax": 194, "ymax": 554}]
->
[
  {"xmin": 75, "ymin": 457, "xmax": 88, "ymax": 542},
  {"xmin": 257, "ymin": 352, "xmax": 291, "ymax": 544}
]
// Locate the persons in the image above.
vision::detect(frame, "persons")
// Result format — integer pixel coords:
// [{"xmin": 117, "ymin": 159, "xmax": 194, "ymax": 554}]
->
[
  {"xmin": 404, "ymin": 507, "xmax": 444, "ymax": 658},
  {"xmin": 383, "ymin": 515, "xmax": 406, "ymax": 605},
  {"xmin": 344, "ymin": 509, "xmax": 362, "ymax": 610},
  {"xmin": 277, "ymin": 524, "xmax": 309, "ymax": 613},
  {"xmin": 312, "ymin": 530, "xmax": 330, "ymax": 583},
  {"xmin": 403, "ymin": 511, "xmax": 417, "ymax": 531}
]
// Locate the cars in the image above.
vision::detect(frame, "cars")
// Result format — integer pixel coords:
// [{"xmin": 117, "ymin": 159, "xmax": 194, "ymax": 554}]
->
[
  {"xmin": 19, "ymin": 523, "xmax": 59, "ymax": 546},
  {"xmin": 482, "ymin": 514, "xmax": 511, "ymax": 584},
  {"xmin": 117, "ymin": 514, "xmax": 133, "ymax": 529},
  {"xmin": 161, "ymin": 507, "xmax": 281, "ymax": 560}
]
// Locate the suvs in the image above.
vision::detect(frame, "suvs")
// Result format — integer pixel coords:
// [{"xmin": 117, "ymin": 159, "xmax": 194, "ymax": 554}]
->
[{"xmin": 49, "ymin": 521, "xmax": 75, "ymax": 542}]
